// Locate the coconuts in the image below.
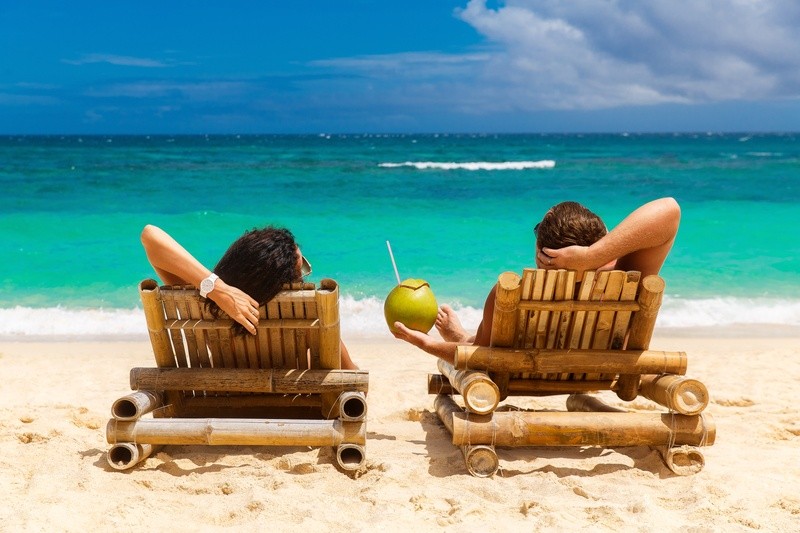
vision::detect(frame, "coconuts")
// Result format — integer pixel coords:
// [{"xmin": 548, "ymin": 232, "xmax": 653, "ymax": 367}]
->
[{"xmin": 384, "ymin": 278, "xmax": 438, "ymax": 335}]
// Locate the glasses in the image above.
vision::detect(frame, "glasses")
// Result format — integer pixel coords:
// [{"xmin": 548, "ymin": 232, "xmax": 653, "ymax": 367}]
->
[{"xmin": 300, "ymin": 255, "xmax": 311, "ymax": 279}]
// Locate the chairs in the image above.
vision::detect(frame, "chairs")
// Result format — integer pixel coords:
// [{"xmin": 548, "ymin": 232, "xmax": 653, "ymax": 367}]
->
[
  {"xmin": 424, "ymin": 267, "xmax": 717, "ymax": 480},
  {"xmin": 106, "ymin": 278, "xmax": 368, "ymax": 472}
]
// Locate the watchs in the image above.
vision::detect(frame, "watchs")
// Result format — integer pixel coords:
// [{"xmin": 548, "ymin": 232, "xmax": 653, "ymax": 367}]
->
[{"xmin": 199, "ymin": 273, "xmax": 219, "ymax": 298}]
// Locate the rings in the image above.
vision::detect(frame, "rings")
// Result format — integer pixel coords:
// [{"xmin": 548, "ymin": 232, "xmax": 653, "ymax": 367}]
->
[{"xmin": 548, "ymin": 258, "xmax": 552, "ymax": 266}]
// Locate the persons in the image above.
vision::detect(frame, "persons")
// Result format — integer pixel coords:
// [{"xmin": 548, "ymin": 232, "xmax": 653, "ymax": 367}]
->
[
  {"xmin": 387, "ymin": 197, "xmax": 681, "ymax": 378},
  {"xmin": 141, "ymin": 221, "xmax": 361, "ymax": 372}
]
